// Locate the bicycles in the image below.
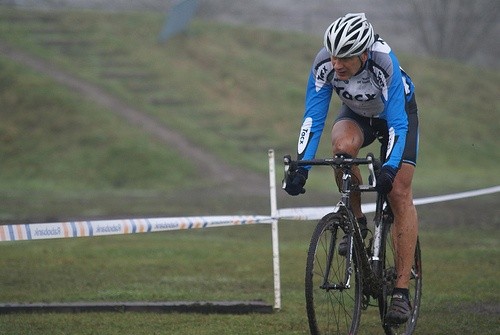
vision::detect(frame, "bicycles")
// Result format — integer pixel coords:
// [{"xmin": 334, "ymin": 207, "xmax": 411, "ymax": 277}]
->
[{"xmin": 280, "ymin": 129, "xmax": 422, "ymax": 335}]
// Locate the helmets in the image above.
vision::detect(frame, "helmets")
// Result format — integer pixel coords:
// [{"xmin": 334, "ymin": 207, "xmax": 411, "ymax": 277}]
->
[{"xmin": 324, "ymin": 13, "xmax": 375, "ymax": 57}]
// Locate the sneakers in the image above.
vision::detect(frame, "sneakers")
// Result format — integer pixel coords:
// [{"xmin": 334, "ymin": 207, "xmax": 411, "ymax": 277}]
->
[
  {"xmin": 339, "ymin": 216, "xmax": 368, "ymax": 255},
  {"xmin": 385, "ymin": 293, "xmax": 412, "ymax": 322}
]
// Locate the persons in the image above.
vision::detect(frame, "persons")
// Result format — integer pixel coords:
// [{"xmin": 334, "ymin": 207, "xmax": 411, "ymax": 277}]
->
[{"xmin": 282, "ymin": 13, "xmax": 419, "ymax": 324}]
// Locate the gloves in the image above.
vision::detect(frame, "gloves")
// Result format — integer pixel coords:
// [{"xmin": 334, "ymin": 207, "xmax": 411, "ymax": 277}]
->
[
  {"xmin": 368, "ymin": 169, "xmax": 393, "ymax": 194},
  {"xmin": 282, "ymin": 171, "xmax": 306, "ymax": 196}
]
